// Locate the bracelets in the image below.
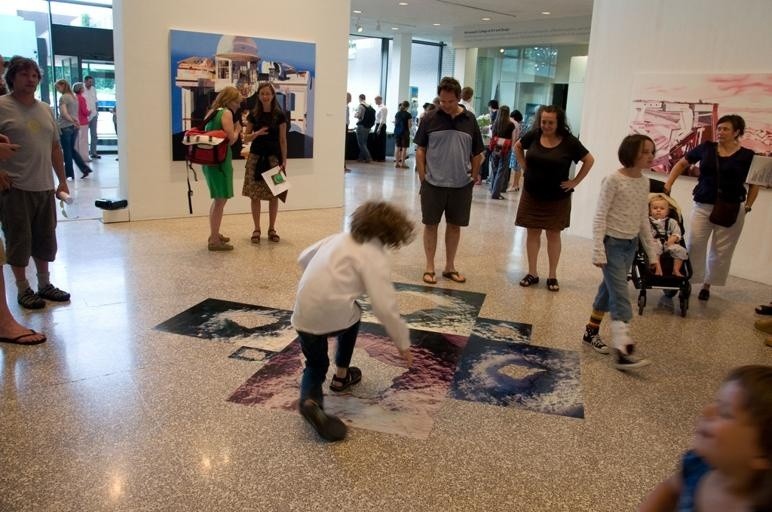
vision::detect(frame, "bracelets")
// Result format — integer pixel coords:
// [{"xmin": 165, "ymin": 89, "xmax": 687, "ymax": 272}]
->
[
  {"xmin": 745, "ymin": 204, "xmax": 751, "ymax": 213},
  {"xmin": 252, "ymin": 131, "xmax": 256, "ymax": 139}
]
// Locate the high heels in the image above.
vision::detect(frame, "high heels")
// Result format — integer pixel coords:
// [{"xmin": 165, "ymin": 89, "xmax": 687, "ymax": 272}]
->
[
  {"xmin": 80, "ymin": 169, "xmax": 94, "ymax": 180},
  {"xmin": 65, "ymin": 174, "xmax": 75, "ymax": 180},
  {"xmin": 506, "ymin": 186, "xmax": 520, "ymax": 192}
]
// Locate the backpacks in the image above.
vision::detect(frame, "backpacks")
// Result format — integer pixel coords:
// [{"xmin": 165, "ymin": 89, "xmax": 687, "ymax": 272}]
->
[{"xmin": 360, "ymin": 103, "xmax": 376, "ymax": 129}]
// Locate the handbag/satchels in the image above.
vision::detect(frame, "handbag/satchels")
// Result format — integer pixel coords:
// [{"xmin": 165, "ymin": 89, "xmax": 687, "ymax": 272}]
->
[
  {"xmin": 179, "ymin": 105, "xmax": 235, "ymax": 167},
  {"xmin": 487, "ymin": 135, "xmax": 512, "ymax": 158}
]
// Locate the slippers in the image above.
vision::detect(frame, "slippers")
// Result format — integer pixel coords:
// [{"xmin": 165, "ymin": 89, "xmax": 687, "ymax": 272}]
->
[
  {"xmin": 441, "ymin": 270, "xmax": 466, "ymax": 282},
  {"xmin": 422, "ymin": 271, "xmax": 438, "ymax": 284},
  {"xmin": 546, "ymin": 277, "xmax": 560, "ymax": 292},
  {"xmin": 0, "ymin": 324, "xmax": 48, "ymax": 346},
  {"xmin": 519, "ymin": 273, "xmax": 540, "ymax": 287}
]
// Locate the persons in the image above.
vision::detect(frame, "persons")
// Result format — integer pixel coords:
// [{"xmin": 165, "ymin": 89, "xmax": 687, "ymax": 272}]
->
[
  {"xmin": 666, "ymin": 114, "xmax": 760, "ymax": 302},
  {"xmin": 243, "ymin": 82, "xmax": 288, "ymax": 243},
  {"xmin": 412, "ymin": 76, "xmax": 484, "ymax": 292},
  {"xmin": 201, "ymin": 87, "xmax": 241, "ymax": 250},
  {"xmin": 344, "ymin": 92, "xmax": 412, "ymax": 172},
  {"xmin": 291, "ymin": 202, "xmax": 419, "ymax": 441},
  {"xmin": 514, "ymin": 105, "xmax": 594, "ymax": 290},
  {"xmin": 0, "ymin": 57, "xmax": 70, "ymax": 346},
  {"xmin": 643, "ymin": 197, "xmax": 688, "ymax": 278},
  {"xmin": 473, "ymin": 100, "xmax": 526, "ymax": 199},
  {"xmin": 580, "ymin": 135, "xmax": 662, "ymax": 368},
  {"xmin": 109, "ymin": 106, "xmax": 119, "ymax": 161},
  {"xmin": 54, "ymin": 76, "xmax": 102, "ymax": 179},
  {"xmin": 636, "ymin": 363, "xmax": 771, "ymax": 512}
]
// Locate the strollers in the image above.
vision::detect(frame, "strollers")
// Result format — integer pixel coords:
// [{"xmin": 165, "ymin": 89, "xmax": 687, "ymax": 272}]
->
[{"xmin": 623, "ymin": 176, "xmax": 693, "ymax": 319}]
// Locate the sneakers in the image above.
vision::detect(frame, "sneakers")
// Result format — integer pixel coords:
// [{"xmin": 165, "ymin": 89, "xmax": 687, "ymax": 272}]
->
[
  {"xmin": 395, "ymin": 164, "xmax": 401, "ymax": 168},
  {"xmin": 400, "ymin": 164, "xmax": 410, "ymax": 169},
  {"xmin": 697, "ymin": 287, "xmax": 711, "ymax": 301},
  {"xmin": 17, "ymin": 287, "xmax": 48, "ymax": 310},
  {"xmin": 763, "ymin": 335, "xmax": 772, "ymax": 347},
  {"xmin": 581, "ymin": 324, "xmax": 611, "ymax": 355},
  {"xmin": 754, "ymin": 317, "xmax": 772, "ymax": 334},
  {"xmin": 613, "ymin": 343, "xmax": 653, "ymax": 371},
  {"xmin": 208, "ymin": 234, "xmax": 231, "ymax": 243},
  {"xmin": 207, "ymin": 241, "xmax": 234, "ymax": 251},
  {"xmin": 37, "ymin": 283, "xmax": 72, "ymax": 303},
  {"xmin": 492, "ymin": 195, "xmax": 505, "ymax": 200}
]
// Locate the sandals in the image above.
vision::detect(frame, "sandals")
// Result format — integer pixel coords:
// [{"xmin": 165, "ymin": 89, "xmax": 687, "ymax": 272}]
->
[
  {"xmin": 755, "ymin": 301, "xmax": 772, "ymax": 318},
  {"xmin": 328, "ymin": 366, "xmax": 363, "ymax": 392},
  {"xmin": 300, "ymin": 398, "xmax": 348, "ymax": 443},
  {"xmin": 268, "ymin": 229, "xmax": 281, "ymax": 242},
  {"xmin": 250, "ymin": 229, "xmax": 262, "ymax": 244}
]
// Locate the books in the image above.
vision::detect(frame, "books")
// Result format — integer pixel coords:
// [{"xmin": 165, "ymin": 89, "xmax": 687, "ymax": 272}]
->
[{"xmin": 261, "ymin": 165, "xmax": 291, "ymax": 196}]
